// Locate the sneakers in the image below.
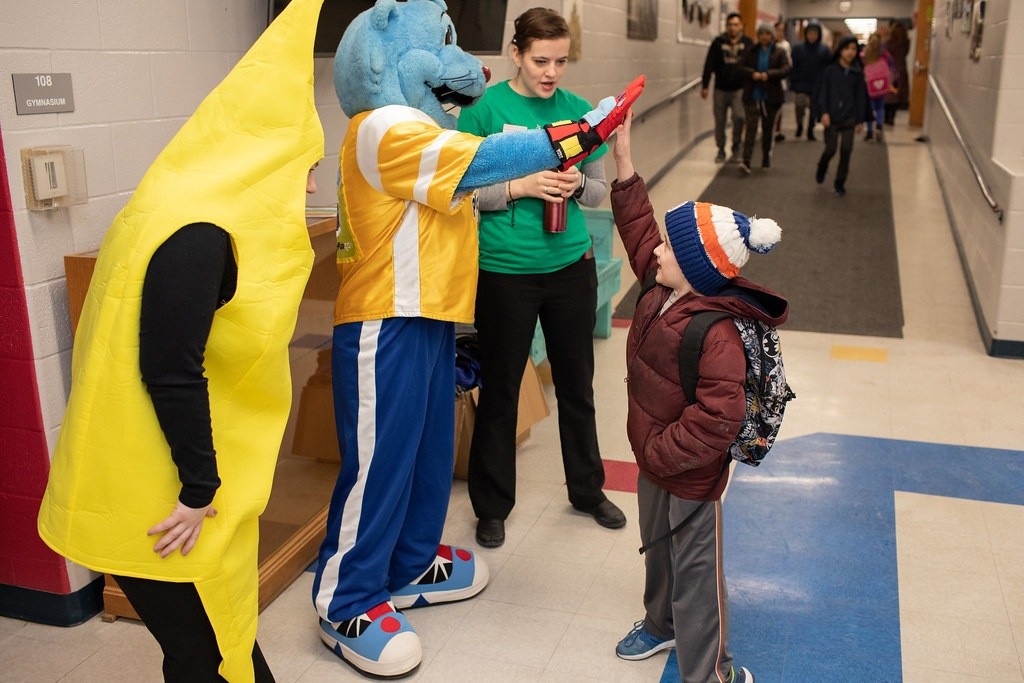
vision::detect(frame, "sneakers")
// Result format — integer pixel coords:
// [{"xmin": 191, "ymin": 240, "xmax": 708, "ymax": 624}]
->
[
  {"xmin": 571, "ymin": 491, "xmax": 626, "ymax": 527},
  {"xmin": 731, "ymin": 665, "xmax": 754, "ymax": 683},
  {"xmin": 616, "ymin": 620, "xmax": 679, "ymax": 659},
  {"xmin": 475, "ymin": 514, "xmax": 506, "ymax": 546}
]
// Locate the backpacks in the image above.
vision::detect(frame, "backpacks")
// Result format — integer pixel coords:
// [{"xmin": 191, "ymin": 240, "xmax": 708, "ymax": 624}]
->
[
  {"xmin": 863, "ymin": 60, "xmax": 890, "ymax": 98},
  {"xmin": 637, "ymin": 268, "xmax": 796, "ymax": 466}
]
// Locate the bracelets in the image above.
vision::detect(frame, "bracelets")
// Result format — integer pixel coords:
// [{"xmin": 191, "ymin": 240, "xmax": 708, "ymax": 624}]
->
[
  {"xmin": 509, "ymin": 181, "xmax": 515, "ymax": 226},
  {"xmin": 581, "ymin": 174, "xmax": 586, "ymax": 189}
]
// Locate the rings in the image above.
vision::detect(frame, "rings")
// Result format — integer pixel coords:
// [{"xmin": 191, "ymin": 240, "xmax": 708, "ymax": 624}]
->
[{"xmin": 545, "ymin": 187, "xmax": 547, "ymax": 193}]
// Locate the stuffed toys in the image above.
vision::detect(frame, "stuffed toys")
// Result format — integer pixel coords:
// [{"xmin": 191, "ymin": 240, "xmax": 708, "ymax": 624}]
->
[{"xmin": 311, "ymin": 0, "xmax": 645, "ymax": 677}]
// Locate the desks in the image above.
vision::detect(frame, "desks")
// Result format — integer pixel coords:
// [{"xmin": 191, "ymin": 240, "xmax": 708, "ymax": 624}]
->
[{"xmin": 615, "ymin": 125, "xmax": 907, "ymax": 339}]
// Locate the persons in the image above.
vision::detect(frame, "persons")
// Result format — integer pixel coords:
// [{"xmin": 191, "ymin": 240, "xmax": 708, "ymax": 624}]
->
[
  {"xmin": 456, "ymin": 7, "xmax": 627, "ymax": 549},
  {"xmin": 738, "ymin": 25, "xmax": 790, "ymax": 175},
  {"xmin": 788, "ymin": 22, "xmax": 831, "ymax": 141},
  {"xmin": 774, "ymin": 22, "xmax": 793, "ymax": 141},
  {"xmin": 38, "ymin": 0, "xmax": 327, "ymax": 683},
  {"xmin": 816, "ymin": 36, "xmax": 877, "ymax": 195},
  {"xmin": 702, "ymin": 13, "xmax": 753, "ymax": 162},
  {"xmin": 610, "ymin": 107, "xmax": 781, "ymax": 683},
  {"xmin": 857, "ymin": 20, "xmax": 910, "ymax": 142}
]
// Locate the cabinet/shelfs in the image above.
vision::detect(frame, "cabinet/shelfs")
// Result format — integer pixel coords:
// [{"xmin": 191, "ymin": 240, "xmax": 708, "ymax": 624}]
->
[{"xmin": 64, "ymin": 215, "xmax": 340, "ymax": 620}]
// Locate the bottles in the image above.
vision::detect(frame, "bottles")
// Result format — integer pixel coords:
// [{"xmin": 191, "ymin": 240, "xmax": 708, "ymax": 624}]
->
[{"xmin": 542, "ymin": 168, "xmax": 570, "ymax": 233}]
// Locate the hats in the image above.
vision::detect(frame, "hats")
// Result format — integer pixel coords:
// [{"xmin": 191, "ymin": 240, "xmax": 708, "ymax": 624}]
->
[
  {"xmin": 666, "ymin": 201, "xmax": 783, "ymax": 297},
  {"xmin": 757, "ymin": 22, "xmax": 777, "ymax": 37}
]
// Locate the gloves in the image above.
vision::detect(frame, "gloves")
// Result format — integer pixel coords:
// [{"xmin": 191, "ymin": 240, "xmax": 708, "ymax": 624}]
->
[{"xmin": 543, "ymin": 74, "xmax": 646, "ymax": 171}]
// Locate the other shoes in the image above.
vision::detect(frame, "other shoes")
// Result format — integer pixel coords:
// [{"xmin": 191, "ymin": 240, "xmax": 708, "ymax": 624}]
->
[
  {"xmin": 739, "ymin": 160, "xmax": 752, "ymax": 174},
  {"xmin": 816, "ymin": 168, "xmax": 825, "ymax": 184},
  {"xmin": 715, "ymin": 151, "xmax": 726, "ymax": 161},
  {"xmin": 834, "ymin": 182, "xmax": 846, "ymax": 195},
  {"xmin": 877, "ymin": 133, "xmax": 882, "ymax": 141},
  {"xmin": 775, "ymin": 134, "xmax": 785, "ymax": 140},
  {"xmin": 864, "ymin": 131, "xmax": 872, "ymax": 139},
  {"xmin": 733, "ymin": 151, "xmax": 744, "ymax": 162},
  {"xmin": 762, "ymin": 161, "xmax": 770, "ymax": 170},
  {"xmin": 807, "ymin": 133, "xmax": 815, "ymax": 139},
  {"xmin": 796, "ymin": 127, "xmax": 802, "ymax": 136}
]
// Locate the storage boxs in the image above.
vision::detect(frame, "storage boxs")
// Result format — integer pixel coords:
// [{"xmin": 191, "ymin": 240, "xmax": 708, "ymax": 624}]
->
[{"xmin": 292, "ymin": 343, "xmax": 550, "ymax": 479}]
